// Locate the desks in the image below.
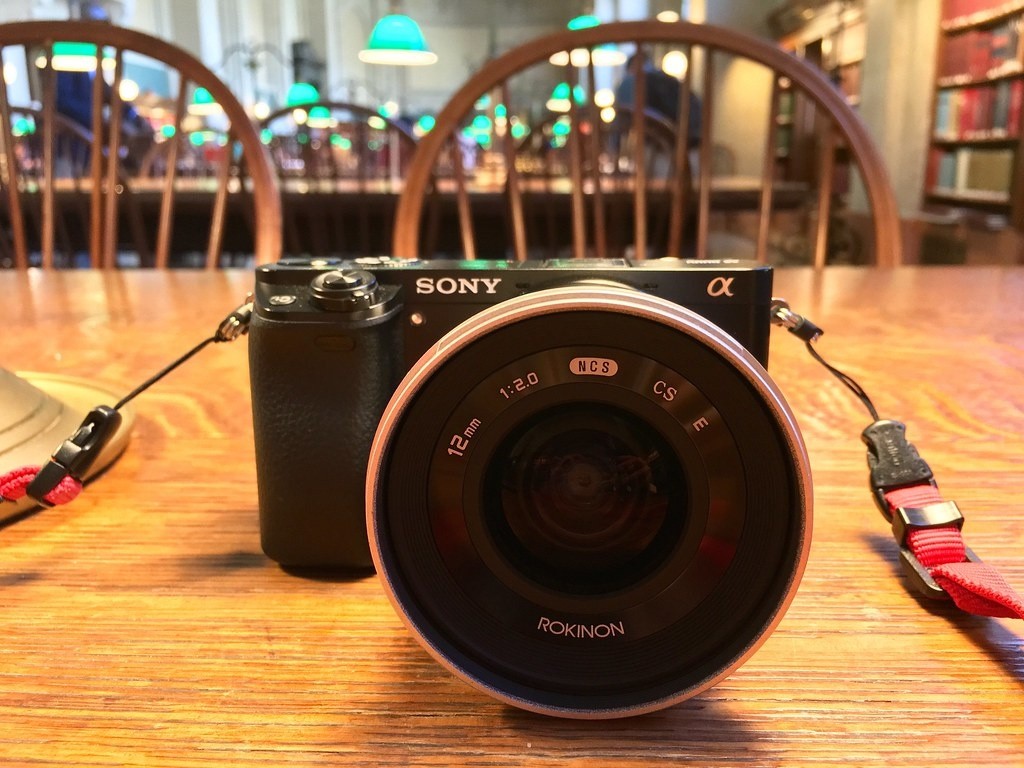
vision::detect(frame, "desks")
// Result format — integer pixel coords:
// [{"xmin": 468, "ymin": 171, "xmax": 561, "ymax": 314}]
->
[{"xmin": 1, "ymin": 253, "xmax": 1023, "ymax": 768}]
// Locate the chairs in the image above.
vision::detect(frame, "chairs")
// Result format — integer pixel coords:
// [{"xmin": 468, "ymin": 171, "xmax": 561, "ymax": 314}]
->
[{"xmin": 0, "ymin": 13, "xmax": 902, "ymax": 266}]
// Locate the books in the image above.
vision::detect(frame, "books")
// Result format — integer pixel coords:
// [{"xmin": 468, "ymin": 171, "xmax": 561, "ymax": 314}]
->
[
  {"xmin": 932, "ymin": 148, "xmax": 1013, "ymax": 199},
  {"xmin": 934, "ymin": 80, "xmax": 1024, "ymax": 130},
  {"xmin": 936, "ymin": 11, "xmax": 1024, "ymax": 77}
]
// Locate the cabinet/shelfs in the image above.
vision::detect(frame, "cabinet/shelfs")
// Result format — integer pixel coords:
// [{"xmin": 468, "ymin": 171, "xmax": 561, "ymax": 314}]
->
[{"xmin": 920, "ymin": 0, "xmax": 1024, "ymax": 226}]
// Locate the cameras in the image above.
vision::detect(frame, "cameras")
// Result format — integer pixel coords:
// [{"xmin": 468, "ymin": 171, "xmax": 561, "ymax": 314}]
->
[{"xmin": 246, "ymin": 253, "xmax": 814, "ymax": 722}]
